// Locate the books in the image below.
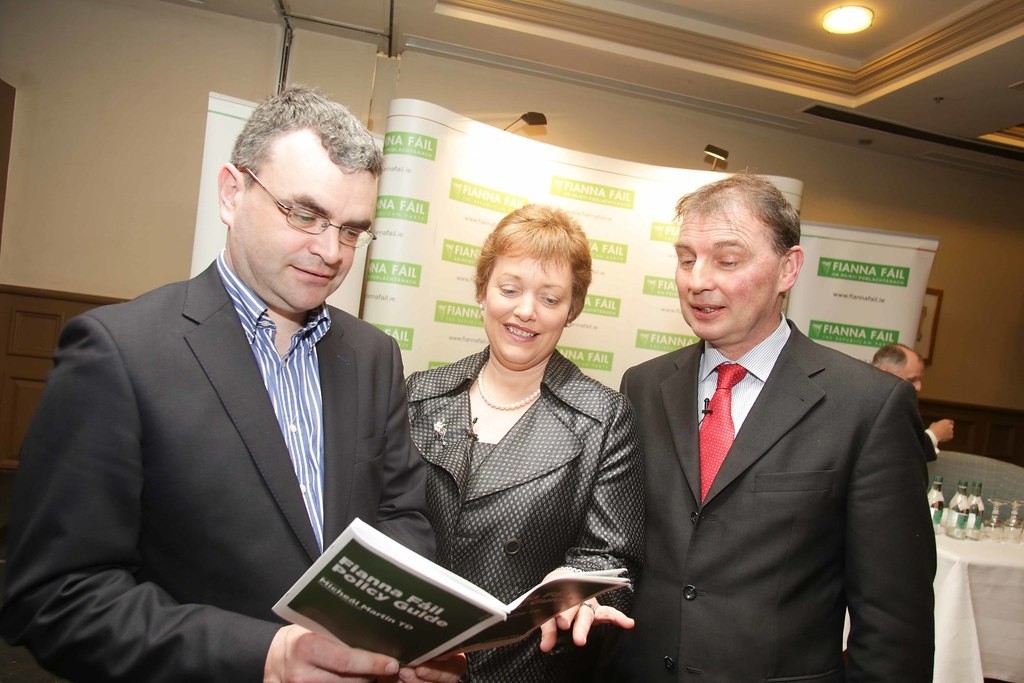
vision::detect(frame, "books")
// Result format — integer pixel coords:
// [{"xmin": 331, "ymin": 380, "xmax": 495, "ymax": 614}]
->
[{"xmin": 271, "ymin": 516, "xmax": 632, "ymax": 666}]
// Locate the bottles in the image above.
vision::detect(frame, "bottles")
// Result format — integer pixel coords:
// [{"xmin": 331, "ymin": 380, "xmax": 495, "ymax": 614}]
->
[
  {"xmin": 946, "ymin": 480, "xmax": 968, "ymax": 540},
  {"xmin": 968, "ymin": 482, "xmax": 984, "ymax": 540},
  {"xmin": 927, "ymin": 476, "xmax": 945, "ymax": 535}
]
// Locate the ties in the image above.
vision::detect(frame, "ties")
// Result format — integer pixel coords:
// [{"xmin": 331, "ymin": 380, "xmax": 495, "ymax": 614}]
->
[{"xmin": 699, "ymin": 364, "xmax": 748, "ymax": 502}]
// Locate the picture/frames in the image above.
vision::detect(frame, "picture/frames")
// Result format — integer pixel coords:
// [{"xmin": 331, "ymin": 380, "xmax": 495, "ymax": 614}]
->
[{"xmin": 914, "ymin": 286, "xmax": 944, "ymax": 366}]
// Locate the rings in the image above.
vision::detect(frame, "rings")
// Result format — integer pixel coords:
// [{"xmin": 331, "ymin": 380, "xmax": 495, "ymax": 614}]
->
[{"xmin": 582, "ymin": 602, "xmax": 595, "ymax": 613}]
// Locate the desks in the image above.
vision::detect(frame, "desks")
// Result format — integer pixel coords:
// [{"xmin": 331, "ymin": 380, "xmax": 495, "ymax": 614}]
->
[{"xmin": 844, "ymin": 509, "xmax": 1024, "ymax": 682}]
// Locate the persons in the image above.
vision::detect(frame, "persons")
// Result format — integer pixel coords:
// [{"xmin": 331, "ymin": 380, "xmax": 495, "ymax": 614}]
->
[
  {"xmin": 871, "ymin": 343, "xmax": 954, "ymax": 463},
  {"xmin": 402, "ymin": 206, "xmax": 643, "ymax": 683},
  {"xmin": 0, "ymin": 82, "xmax": 467, "ymax": 683},
  {"xmin": 618, "ymin": 171, "xmax": 938, "ymax": 682}
]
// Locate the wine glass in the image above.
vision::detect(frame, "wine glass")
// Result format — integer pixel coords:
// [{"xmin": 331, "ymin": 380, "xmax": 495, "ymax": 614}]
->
[
  {"xmin": 1002, "ymin": 500, "xmax": 1024, "ymax": 540},
  {"xmin": 980, "ymin": 498, "xmax": 1008, "ymax": 542}
]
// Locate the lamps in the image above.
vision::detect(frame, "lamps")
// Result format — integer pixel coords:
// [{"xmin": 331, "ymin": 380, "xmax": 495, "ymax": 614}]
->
[
  {"xmin": 704, "ymin": 144, "xmax": 728, "ymax": 171},
  {"xmin": 504, "ymin": 112, "xmax": 547, "ymax": 131}
]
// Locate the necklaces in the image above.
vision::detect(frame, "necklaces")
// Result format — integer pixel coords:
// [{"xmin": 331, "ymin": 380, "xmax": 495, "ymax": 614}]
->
[{"xmin": 476, "ymin": 362, "xmax": 541, "ymax": 410}]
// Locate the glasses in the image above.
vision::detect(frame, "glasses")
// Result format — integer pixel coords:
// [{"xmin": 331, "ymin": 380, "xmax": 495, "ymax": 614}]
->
[{"xmin": 239, "ymin": 167, "xmax": 377, "ymax": 248}]
